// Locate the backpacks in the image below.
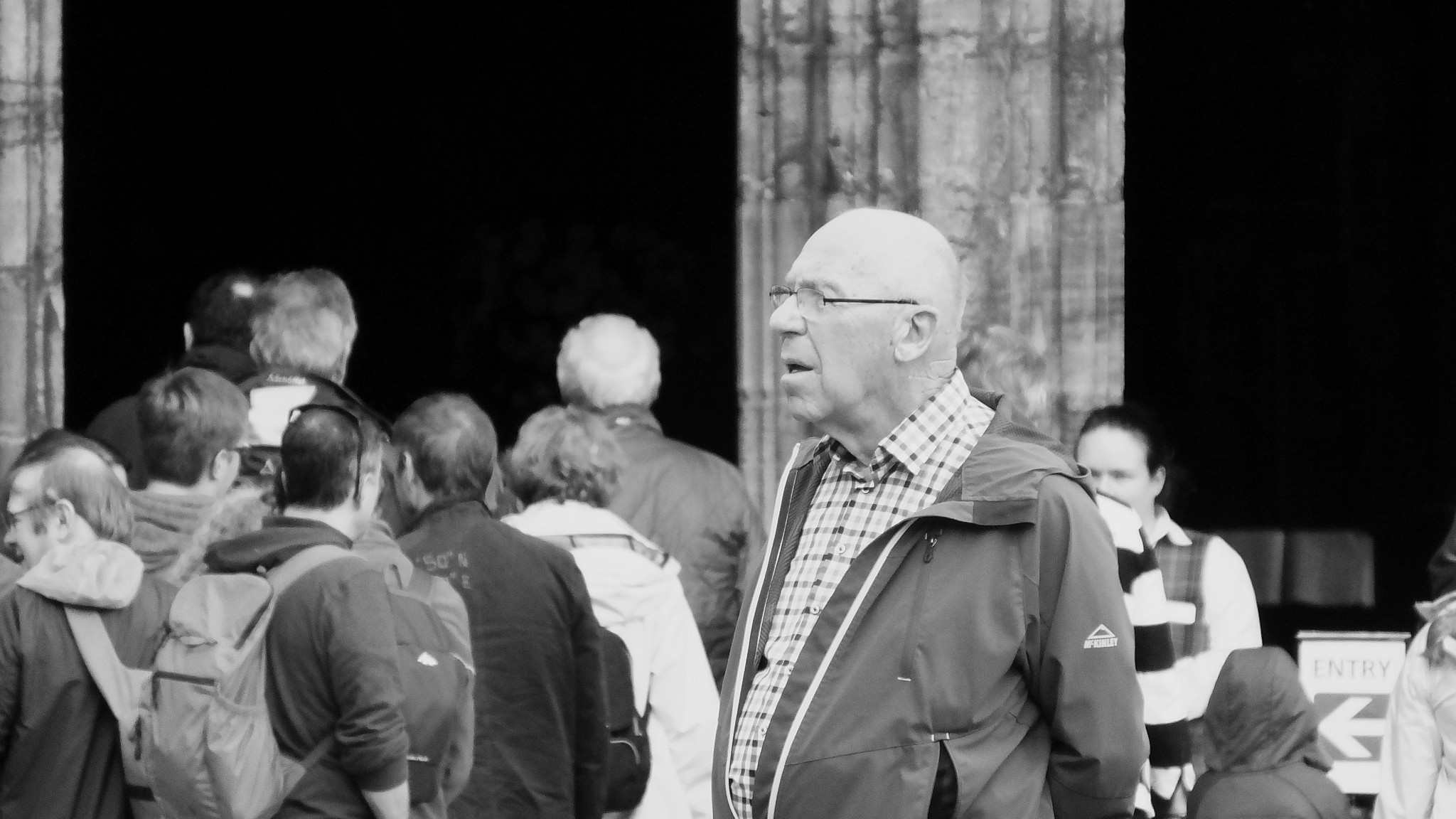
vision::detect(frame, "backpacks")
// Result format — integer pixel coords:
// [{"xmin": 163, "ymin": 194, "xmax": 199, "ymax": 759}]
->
[
  {"xmin": 66, "ymin": 603, "xmax": 161, "ymax": 819},
  {"xmin": 141, "ymin": 545, "xmax": 360, "ymax": 819},
  {"xmin": 600, "ymin": 627, "xmax": 651, "ymax": 813}
]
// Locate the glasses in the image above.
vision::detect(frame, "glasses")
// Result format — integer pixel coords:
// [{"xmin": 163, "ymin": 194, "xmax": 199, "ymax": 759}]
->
[
  {"xmin": 769, "ymin": 284, "xmax": 920, "ymax": 316},
  {"xmin": 289, "ymin": 404, "xmax": 365, "ymax": 499}
]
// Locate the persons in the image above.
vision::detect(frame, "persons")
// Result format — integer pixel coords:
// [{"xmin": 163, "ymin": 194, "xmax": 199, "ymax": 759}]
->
[
  {"xmin": 0, "ymin": 270, "xmax": 769, "ymax": 819},
  {"xmin": 1073, "ymin": 405, "xmax": 1264, "ymax": 819},
  {"xmin": 1372, "ymin": 525, "xmax": 1456, "ymax": 819},
  {"xmin": 1095, "ymin": 488, "xmax": 1196, "ymax": 819},
  {"xmin": 711, "ymin": 207, "xmax": 1151, "ymax": 819},
  {"xmin": 1187, "ymin": 648, "xmax": 1352, "ymax": 819}
]
